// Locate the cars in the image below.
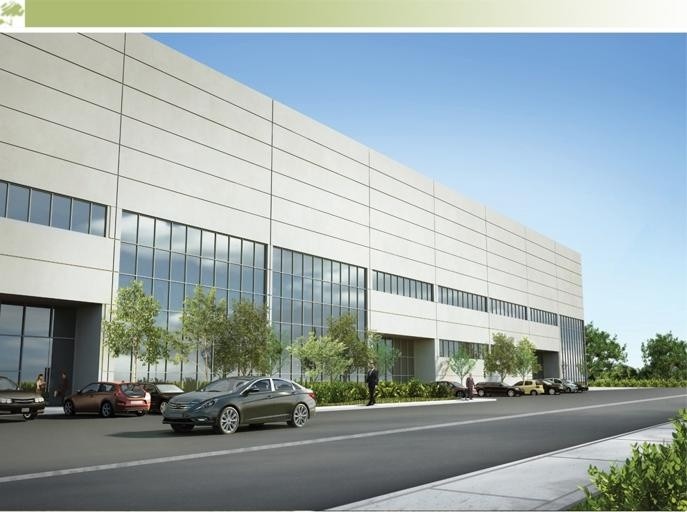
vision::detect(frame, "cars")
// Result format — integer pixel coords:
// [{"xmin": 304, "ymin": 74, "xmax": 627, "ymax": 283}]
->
[
  {"xmin": 162, "ymin": 375, "xmax": 317, "ymax": 434},
  {"xmin": 426, "ymin": 377, "xmax": 588, "ymax": 399},
  {"xmin": 0, "ymin": 376, "xmax": 190, "ymax": 421}
]
